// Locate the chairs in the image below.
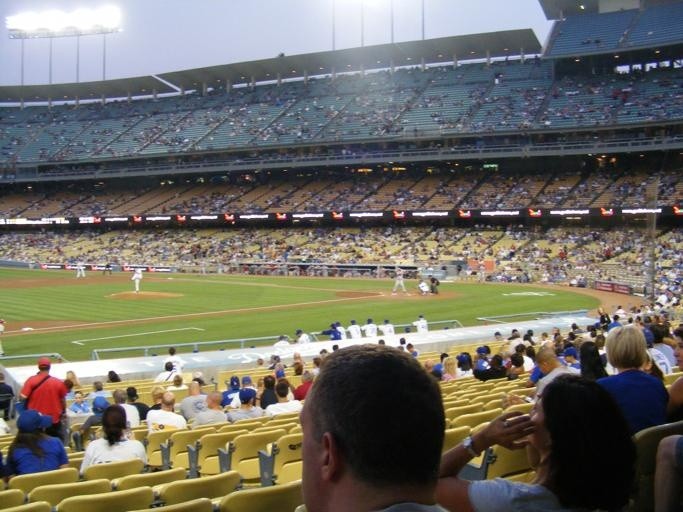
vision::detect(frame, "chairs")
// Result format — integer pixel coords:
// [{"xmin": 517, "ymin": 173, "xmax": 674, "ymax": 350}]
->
[
  {"xmin": 1, "ymin": 5, "xmax": 682, "ymax": 293},
  {"xmin": 0, "ymin": 328, "xmax": 683, "ymax": 512}
]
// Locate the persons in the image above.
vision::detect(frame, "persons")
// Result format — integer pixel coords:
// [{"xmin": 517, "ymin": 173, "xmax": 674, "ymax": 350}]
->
[
  {"xmin": 359, "ymin": 317, "xmax": 376, "ymax": 337},
  {"xmin": 412, "ymin": 314, "xmax": 427, "ymax": 332},
  {"xmin": 335, "ymin": 321, "xmax": 346, "ymax": 339},
  {"xmin": 391, "ymin": 268, "xmax": 407, "ymax": 295},
  {"xmin": 489, "ymin": 279, "xmax": 682, "ymax": 341},
  {"xmin": 404, "ymin": 327, "xmax": 410, "ymax": 333},
  {"xmin": 273, "ymin": 334, "xmax": 289, "ymax": 346},
  {"xmin": 130, "ymin": 269, "xmax": 142, "ymax": 294},
  {"xmin": 346, "ymin": 320, "xmax": 361, "ymax": 338},
  {"xmin": 319, "ymin": 323, "xmax": 341, "ymax": 340},
  {"xmin": 295, "ymin": 329, "xmax": 309, "ymax": 343},
  {"xmin": 416, "ymin": 280, "xmax": 428, "ymax": 296},
  {"xmin": 428, "ymin": 275, "xmax": 440, "ymax": 294},
  {"xmin": 377, "ymin": 319, "xmax": 394, "ymax": 335}
]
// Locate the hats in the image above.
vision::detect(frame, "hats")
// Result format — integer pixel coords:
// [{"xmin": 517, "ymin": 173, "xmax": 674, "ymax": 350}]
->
[
  {"xmin": 92, "ymin": 395, "xmax": 111, "ymax": 417},
  {"xmin": 278, "ymin": 328, "xmax": 301, "ymax": 341},
  {"xmin": 431, "ymin": 346, "xmax": 488, "ymax": 376},
  {"xmin": 558, "ymin": 347, "xmax": 577, "ymax": 357},
  {"xmin": 643, "ymin": 329, "xmax": 655, "ymax": 345},
  {"xmin": 16, "ymin": 410, "xmax": 54, "ymax": 430},
  {"xmin": 38, "ymin": 358, "xmax": 51, "ymax": 368},
  {"xmin": 330, "ymin": 319, "xmax": 391, "ymax": 329},
  {"xmin": 230, "ymin": 368, "xmax": 285, "ymax": 403}
]
